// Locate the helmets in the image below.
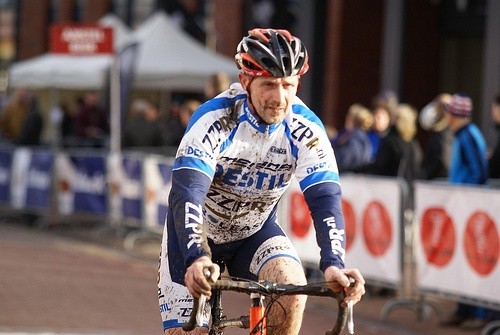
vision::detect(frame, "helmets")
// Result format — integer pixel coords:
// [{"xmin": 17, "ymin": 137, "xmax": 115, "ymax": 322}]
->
[{"xmin": 235, "ymin": 29, "xmax": 309, "ymax": 77}]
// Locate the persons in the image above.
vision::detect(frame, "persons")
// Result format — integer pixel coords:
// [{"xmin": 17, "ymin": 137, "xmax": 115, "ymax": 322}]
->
[
  {"xmin": 157, "ymin": 29, "xmax": 366, "ymax": 334},
  {"xmin": 2, "ymin": 70, "xmax": 500, "ymax": 327}
]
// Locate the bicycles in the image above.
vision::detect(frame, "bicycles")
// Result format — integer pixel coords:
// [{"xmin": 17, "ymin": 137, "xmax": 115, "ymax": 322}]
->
[{"xmin": 182, "ymin": 269, "xmax": 357, "ymax": 334}]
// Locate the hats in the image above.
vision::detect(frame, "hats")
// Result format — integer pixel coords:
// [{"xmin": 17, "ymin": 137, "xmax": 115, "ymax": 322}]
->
[{"xmin": 445, "ymin": 95, "xmax": 473, "ymax": 115}]
